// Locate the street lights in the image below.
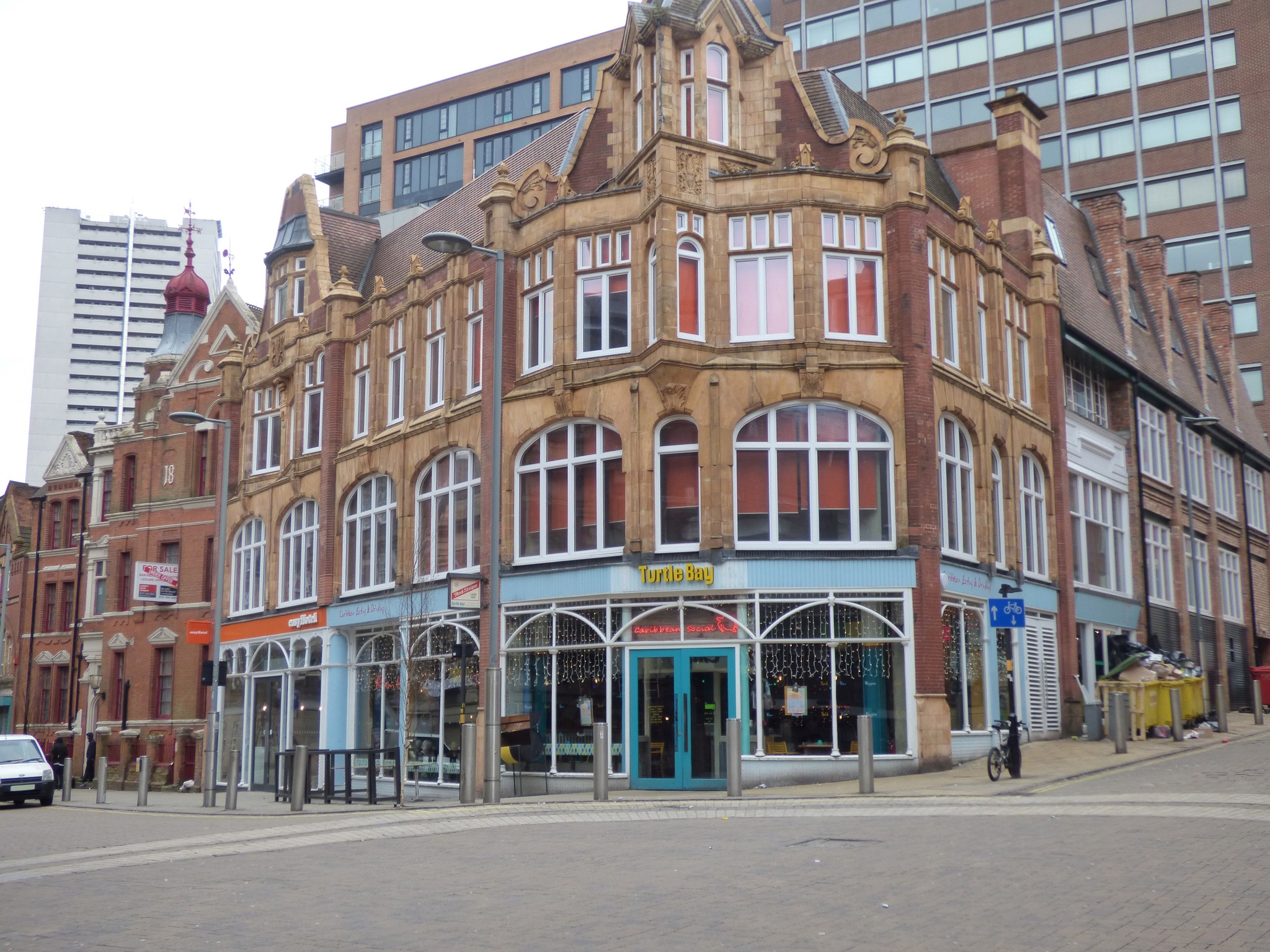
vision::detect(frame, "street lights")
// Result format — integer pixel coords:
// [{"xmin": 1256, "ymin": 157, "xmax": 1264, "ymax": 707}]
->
[
  {"xmin": 167, "ymin": 411, "xmax": 231, "ymax": 807},
  {"xmin": 421, "ymin": 231, "xmax": 501, "ymax": 805}
]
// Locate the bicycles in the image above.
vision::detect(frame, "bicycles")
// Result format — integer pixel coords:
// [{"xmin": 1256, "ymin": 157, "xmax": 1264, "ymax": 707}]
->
[{"xmin": 987, "ymin": 720, "xmax": 1030, "ymax": 782}]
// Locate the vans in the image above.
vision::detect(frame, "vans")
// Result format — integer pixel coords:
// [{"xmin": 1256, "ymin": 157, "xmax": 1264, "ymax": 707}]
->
[{"xmin": 0, "ymin": 733, "xmax": 56, "ymax": 806}]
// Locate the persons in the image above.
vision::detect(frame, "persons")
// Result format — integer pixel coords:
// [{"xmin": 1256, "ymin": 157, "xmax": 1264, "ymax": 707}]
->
[
  {"xmin": 80, "ymin": 732, "xmax": 96, "ymax": 786},
  {"xmin": 51, "ymin": 737, "xmax": 68, "ymax": 790}
]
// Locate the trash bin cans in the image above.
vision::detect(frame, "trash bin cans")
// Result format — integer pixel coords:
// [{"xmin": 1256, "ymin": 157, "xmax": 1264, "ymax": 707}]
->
[
  {"xmin": 1249, "ymin": 665, "xmax": 1270, "ymax": 714},
  {"xmin": 1098, "ymin": 677, "xmax": 1205, "ymax": 738},
  {"xmin": 1084, "ymin": 701, "xmax": 1101, "ymax": 741}
]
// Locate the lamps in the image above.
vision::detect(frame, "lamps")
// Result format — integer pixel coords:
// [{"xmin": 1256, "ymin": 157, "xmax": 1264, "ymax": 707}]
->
[{"xmin": 89, "ymin": 675, "xmax": 106, "ymax": 700}]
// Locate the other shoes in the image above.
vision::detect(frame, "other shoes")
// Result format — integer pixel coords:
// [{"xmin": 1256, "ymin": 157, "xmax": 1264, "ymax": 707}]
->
[
  {"xmin": 54, "ymin": 788, "xmax": 58, "ymax": 791},
  {"xmin": 59, "ymin": 787, "xmax": 62, "ymax": 790},
  {"xmin": 87, "ymin": 780, "xmax": 92, "ymax": 782},
  {"xmin": 78, "ymin": 778, "xmax": 85, "ymax": 781}
]
